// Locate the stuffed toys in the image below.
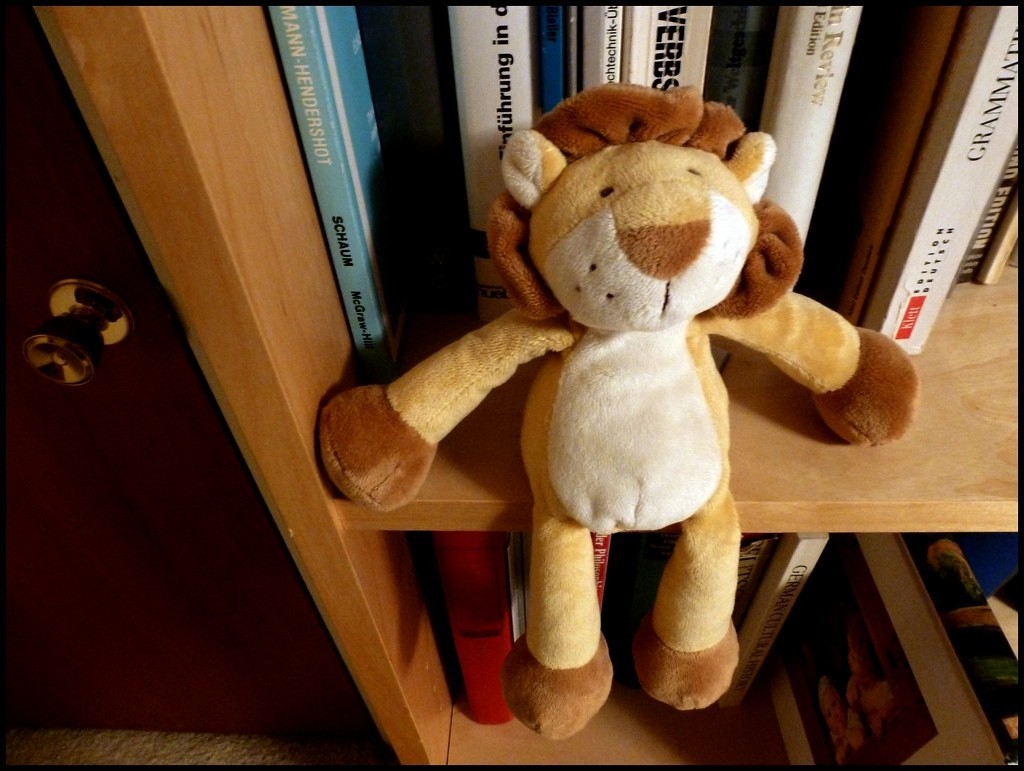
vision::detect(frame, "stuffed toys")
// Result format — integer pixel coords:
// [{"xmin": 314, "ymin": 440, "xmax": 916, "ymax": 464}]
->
[{"xmin": 317, "ymin": 80, "xmax": 920, "ymax": 736}]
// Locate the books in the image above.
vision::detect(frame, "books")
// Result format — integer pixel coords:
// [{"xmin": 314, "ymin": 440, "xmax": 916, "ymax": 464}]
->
[{"xmin": 266, "ymin": 2, "xmax": 1018, "ymax": 354}]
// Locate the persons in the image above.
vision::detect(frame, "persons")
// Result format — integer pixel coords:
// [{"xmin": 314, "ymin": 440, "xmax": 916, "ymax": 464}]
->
[
  {"xmin": 430, "ymin": 528, "xmax": 1021, "ymax": 769},
  {"xmin": 845, "ymin": 618, "xmax": 902, "ymax": 736},
  {"xmin": 817, "ymin": 674, "xmax": 866, "ymax": 767}
]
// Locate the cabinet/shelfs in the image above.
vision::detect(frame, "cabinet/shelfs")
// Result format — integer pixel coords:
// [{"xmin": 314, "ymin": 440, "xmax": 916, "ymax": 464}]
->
[{"xmin": 33, "ymin": 6, "xmax": 1020, "ymax": 765}]
